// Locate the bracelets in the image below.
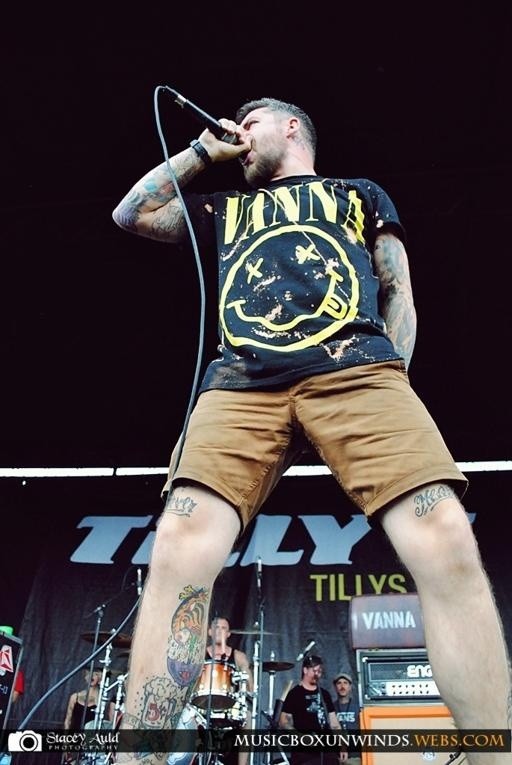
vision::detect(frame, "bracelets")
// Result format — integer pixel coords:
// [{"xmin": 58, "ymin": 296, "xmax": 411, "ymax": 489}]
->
[{"xmin": 189, "ymin": 139, "xmax": 213, "ymax": 168}]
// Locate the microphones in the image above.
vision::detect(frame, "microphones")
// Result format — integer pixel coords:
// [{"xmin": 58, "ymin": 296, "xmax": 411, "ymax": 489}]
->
[
  {"xmin": 165, "ymin": 84, "xmax": 239, "ymax": 146},
  {"xmin": 297, "ymin": 641, "xmax": 316, "ymax": 661},
  {"xmin": 256, "ymin": 557, "xmax": 263, "ymax": 597},
  {"xmin": 135, "ymin": 568, "xmax": 143, "ymax": 595}
]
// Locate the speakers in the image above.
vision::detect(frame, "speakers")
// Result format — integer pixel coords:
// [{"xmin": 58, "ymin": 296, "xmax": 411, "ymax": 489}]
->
[{"xmin": 358, "ymin": 708, "xmax": 471, "ymax": 765}]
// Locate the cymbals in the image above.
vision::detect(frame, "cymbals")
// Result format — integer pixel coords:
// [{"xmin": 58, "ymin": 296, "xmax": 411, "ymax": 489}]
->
[
  {"xmin": 249, "ymin": 661, "xmax": 295, "ymax": 671},
  {"xmin": 80, "ymin": 629, "xmax": 132, "ymax": 647},
  {"xmin": 229, "ymin": 629, "xmax": 276, "ymax": 635}
]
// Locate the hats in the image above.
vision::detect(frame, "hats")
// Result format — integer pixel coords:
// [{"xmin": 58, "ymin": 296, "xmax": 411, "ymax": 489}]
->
[
  {"xmin": 83, "ymin": 658, "xmax": 111, "ymax": 674},
  {"xmin": 334, "ymin": 673, "xmax": 352, "ymax": 684}
]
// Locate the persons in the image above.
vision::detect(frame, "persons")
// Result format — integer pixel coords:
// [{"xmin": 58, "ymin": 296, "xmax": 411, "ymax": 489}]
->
[
  {"xmin": 11, "ymin": 671, "xmax": 25, "ymax": 703},
  {"xmin": 63, "ymin": 619, "xmax": 361, "ymax": 764},
  {"xmin": 111, "ymin": 96, "xmax": 510, "ymax": 765}
]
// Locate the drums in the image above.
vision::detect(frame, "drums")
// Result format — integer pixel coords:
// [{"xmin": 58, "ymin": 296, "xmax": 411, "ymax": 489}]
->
[
  {"xmin": 201, "ymin": 714, "xmax": 245, "ymax": 765},
  {"xmin": 190, "ymin": 659, "xmax": 238, "ymax": 709},
  {"xmin": 192, "ymin": 708, "xmax": 221, "ymax": 765}
]
